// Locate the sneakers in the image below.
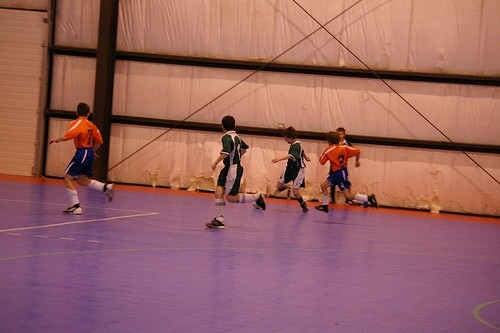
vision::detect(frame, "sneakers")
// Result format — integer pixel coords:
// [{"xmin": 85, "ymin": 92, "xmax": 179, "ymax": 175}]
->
[
  {"xmin": 62, "ymin": 203, "xmax": 82, "ymax": 215},
  {"xmin": 255, "ymin": 191, "xmax": 267, "ymax": 211},
  {"xmin": 205, "ymin": 217, "xmax": 225, "ymax": 229},
  {"xmin": 103, "ymin": 183, "xmax": 114, "ymax": 202}
]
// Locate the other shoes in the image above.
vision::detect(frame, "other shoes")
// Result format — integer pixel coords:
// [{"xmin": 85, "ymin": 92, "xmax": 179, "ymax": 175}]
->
[
  {"xmin": 300, "ymin": 202, "xmax": 309, "ymax": 213},
  {"xmin": 278, "ymin": 184, "xmax": 288, "ymax": 191},
  {"xmin": 314, "ymin": 204, "xmax": 329, "ymax": 213},
  {"xmin": 367, "ymin": 193, "xmax": 377, "ymax": 209}
]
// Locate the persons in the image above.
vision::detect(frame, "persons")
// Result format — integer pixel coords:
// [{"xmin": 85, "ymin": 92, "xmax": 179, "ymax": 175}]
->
[
  {"xmin": 314, "ymin": 129, "xmax": 380, "ymax": 212},
  {"xmin": 328, "ymin": 127, "xmax": 355, "ymax": 207},
  {"xmin": 205, "ymin": 114, "xmax": 267, "ymax": 229},
  {"xmin": 48, "ymin": 101, "xmax": 115, "ymax": 215},
  {"xmin": 270, "ymin": 126, "xmax": 313, "ymax": 213}
]
